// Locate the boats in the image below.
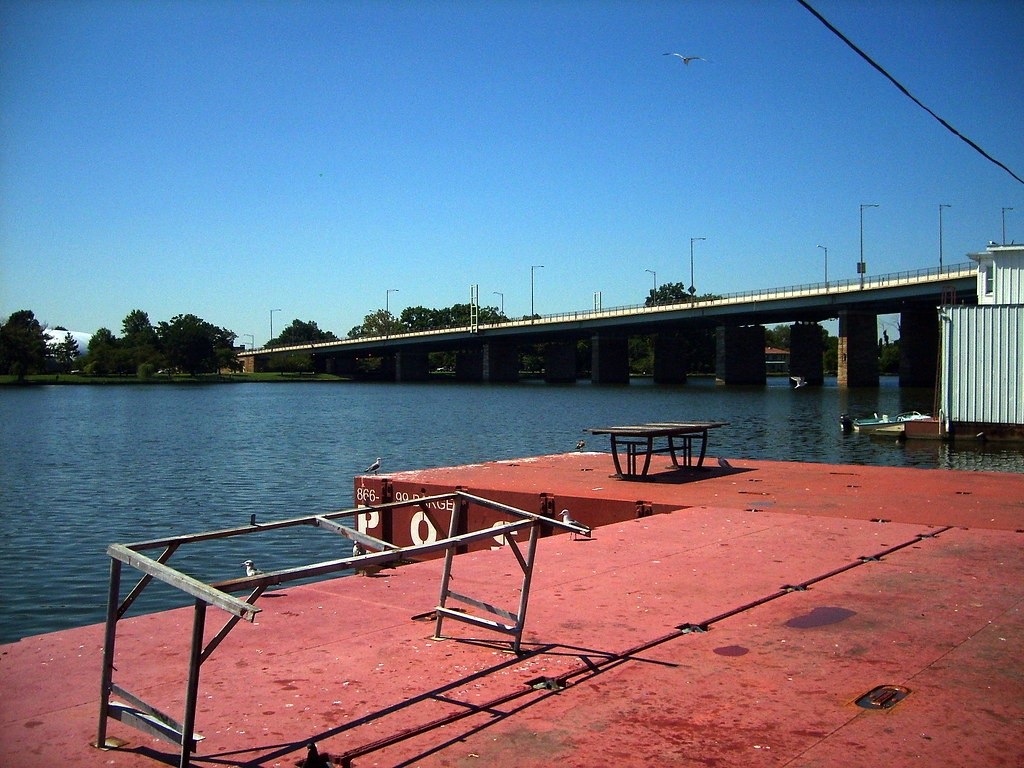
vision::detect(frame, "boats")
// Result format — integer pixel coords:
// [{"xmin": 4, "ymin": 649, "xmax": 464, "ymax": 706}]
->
[{"xmin": 852, "ymin": 410, "xmax": 931, "ymax": 436}]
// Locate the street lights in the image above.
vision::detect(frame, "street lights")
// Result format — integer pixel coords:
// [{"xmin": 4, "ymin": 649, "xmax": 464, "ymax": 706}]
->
[
  {"xmin": 386, "ymin": 288, "xmax": 400, "ymax": 339},
  {"xmin": 644, "ymin": 268, "xmax": 656, "ymax": 305},
  {"xmin": 690, "ymin": 237, "xmax": 706, "ymax": 309},
  {"xmin": 1000, "ymin": 207, "xmax": 1014, "ymax": 247},
  {"xmin": 492, "ymin": 291, "xmax": 504, "ymax": 315},
  {"xmin": 816, "ymin": 244, "xmax": 828, "ymax": 288},
  {"xmin": 859, "ymin": 203, "xmax": 879, "ymax": 288},
  {"xmin": 530, "ymin": 265, "xmax": 545, "ymax": 325},
  {"xmin": 243, "ymin": 333, "xmax": 254, "ymax": 351},
  {"xmin": 271, "ymin": 308, "xmax": 282, "ymax": 352},
  {"xmin": 938, "ymin": 203, "xmax": 952, "ymax": 274}
]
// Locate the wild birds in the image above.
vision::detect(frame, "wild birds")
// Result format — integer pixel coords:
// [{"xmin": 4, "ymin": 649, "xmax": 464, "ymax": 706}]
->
[
  {"xmin": 575, "ymin": 440, "xmax": 585, "ymax": 453},
  {"xmin": 989, "ymin": 240, "xmax": 996, "ymax": 245},
  {"xmin": 241, "ymin": 560, "xmax": 282, "ymax": 587},
  {"xmin": 791, "ymin": 377, "xmax": 808, "ymax": 388},
  {"xmin": 364, "ymin": 458, "xmax": 382, "ymax": 476},
  {"xmin": 558, "ymin": 509, "xmax": 592, "ymax": 541},
  {"xmin": 353, "ymin": 541, "xmax": 366, "ymax": 557},
  {"xmin": 717, "ymin": 456, "xmax": 735, "ymax": 474},
  {"xmin": 662, "ymin": 53, "xmax": 707, "ymax": 66}
]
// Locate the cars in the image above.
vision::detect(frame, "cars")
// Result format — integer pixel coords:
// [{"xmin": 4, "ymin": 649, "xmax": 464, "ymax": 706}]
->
[{"xmin": 157, "ymin": 368, "xmax": 172, "ymax": 374}]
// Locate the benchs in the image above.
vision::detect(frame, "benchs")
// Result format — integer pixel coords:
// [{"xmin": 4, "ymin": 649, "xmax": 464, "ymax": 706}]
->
[{"xmin": 583, "ymin": 422, "xmax": 730, "ymax": 482}]
